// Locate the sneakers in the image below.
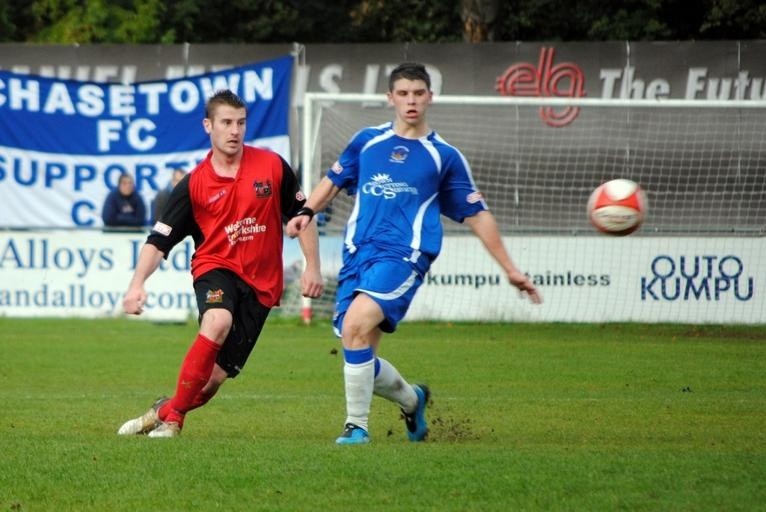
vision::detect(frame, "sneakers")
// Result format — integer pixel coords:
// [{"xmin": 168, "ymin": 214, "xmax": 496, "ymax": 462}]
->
[
  {"xmin": 401, "ymin": 382, "xmax": 431, "ymax": 442},
  {"xmin": 146, "ymin": 420, "xmax": 182, "ymax": 438},
  {"xmin": 116, "ymin": 395, "xmax": 170, "ymax": 436},
  {"xmin": 335, "ymin": 421, "xmax": 371, "ymax": 445}
]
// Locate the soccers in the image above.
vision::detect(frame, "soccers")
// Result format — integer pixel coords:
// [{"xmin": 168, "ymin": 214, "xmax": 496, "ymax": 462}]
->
[{"xmin": 587, "ymin": 177, "xmax": 648, "ymax": 236}]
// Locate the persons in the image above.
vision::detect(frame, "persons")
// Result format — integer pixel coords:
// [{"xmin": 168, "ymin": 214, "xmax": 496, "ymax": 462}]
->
[
  {"xmin": 110, "ymin": 87, "xmax": 326, "ymax": 441},
  {"xmin": 150, "ymin": 167, "xmax": 194, "ymax": 219},
  {"xmin": 101, "ymin": 172, "xmax": 145, "ymax": 229},
  {"xmin": 278, "ymin": 62, "xmax": 545, "ymax": 445}
]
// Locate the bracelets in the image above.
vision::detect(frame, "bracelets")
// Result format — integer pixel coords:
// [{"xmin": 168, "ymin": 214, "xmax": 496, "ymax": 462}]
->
[{"xmin": 295, "ymin": 206, "xmax": 315, "ymax": 221}]
